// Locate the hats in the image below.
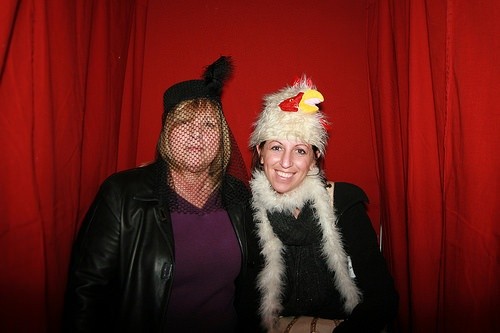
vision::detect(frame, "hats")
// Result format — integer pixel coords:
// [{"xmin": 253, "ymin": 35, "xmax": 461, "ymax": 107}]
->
[
  {"xmin": 247, "ymin": 73, "xmax": 332, "ymax": 156},
  {"xmin": 161, "ymin": 55, "xmax": 234, "ymax": 120}
]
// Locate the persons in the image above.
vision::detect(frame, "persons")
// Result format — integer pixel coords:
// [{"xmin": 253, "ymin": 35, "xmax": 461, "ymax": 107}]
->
[
  {"xmin": 246, "ymin": 71, "xmax": 402, "ymax": 332},
  {"xmin": 56, "ymin": 55, "xmax": 252, "ymax": 332}
]
